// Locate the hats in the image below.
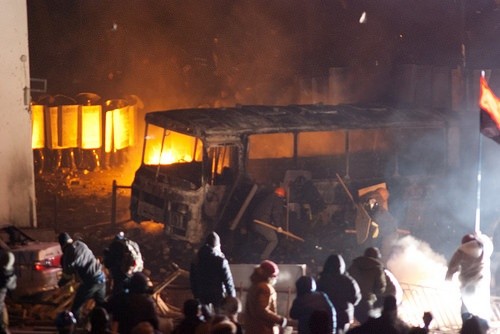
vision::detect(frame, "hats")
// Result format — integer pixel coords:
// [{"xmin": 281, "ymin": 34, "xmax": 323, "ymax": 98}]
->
[
  {"xmin": 206, "ymin": 231, "xmax": 221, "ymax": 249},
  {"xmin": 182, "ymin": 299, "xmax": 204, "ymax": 312},
  {"xmin": 58, "ymin": 232, "xmax": 71, "ymax": 248},
  {"xmin": 461, "ymin": 234, "xmax": 478, "ymax": 244},
  {"xmin": 364, "ymin": 246, "xmax": 382, "ymax": 259},
  {"xmin": 254, "ymin": 260, "xmax": 281, "ymax": 278}
]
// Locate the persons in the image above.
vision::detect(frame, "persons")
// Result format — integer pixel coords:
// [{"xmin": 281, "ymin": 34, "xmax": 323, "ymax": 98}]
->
[
  {"xmin": 238, "ymin": 261, "xmax": 288, "ymax": 334},
  {"xmin": 444, "ymin": 232, "xmax": 494, "ymax": 325},
  {"xmin": 210, "ymin": 165, "xmax": 400, "ymax": 262},
  {"xmin": 189, "ymin": 230, "xmax": 235, "ymax": 323},
  {"xmin": 346, "ymin": 247, "xmax": 388, "ymax": 324},
  {"xmin": 313, "ymin": 253, "xmax": 363, "ymax": 334},
  {"xmin": 55, "ymin": 277, "xmax": 490, "ymax": 334},
  {"xmin": 0, "ymin": 239, "xmax": 17, "ymax": 334},
  {"xmin": 98, "ymin": 231, "xmax": 143, "ymax": 297},
  {"xmin": 56, "ymin": 231, "xmax": 108, "ymax": 319},
  {"xmin": 288, "ymin": 276, "xmax": 337, "ymax": 334}
]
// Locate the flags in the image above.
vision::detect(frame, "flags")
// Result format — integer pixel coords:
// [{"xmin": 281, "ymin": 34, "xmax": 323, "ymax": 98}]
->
[{"xmin": 478, "ymin": 76, "xmax": 500, "ymax": 145}]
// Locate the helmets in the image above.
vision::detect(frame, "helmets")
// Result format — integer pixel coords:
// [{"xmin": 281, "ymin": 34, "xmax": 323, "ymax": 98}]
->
[
  {"xmin": 273, "ymin": 187, "xmax": 286, "ymax": 199},
  {"xmin": 52, "ymin": 309, "xmax": 76, "ymax": 329}
]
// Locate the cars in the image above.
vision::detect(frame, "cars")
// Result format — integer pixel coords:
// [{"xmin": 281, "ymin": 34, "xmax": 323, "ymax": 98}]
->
[{"xmin": 0, "ymin": 223, "xmax": 68, "ymax": 298}]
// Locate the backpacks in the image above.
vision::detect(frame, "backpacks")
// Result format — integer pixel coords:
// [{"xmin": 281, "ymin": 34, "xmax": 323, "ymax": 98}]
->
[{"xmin": 114, "ymin": 238, "xmax": 146, "ymax": 278}]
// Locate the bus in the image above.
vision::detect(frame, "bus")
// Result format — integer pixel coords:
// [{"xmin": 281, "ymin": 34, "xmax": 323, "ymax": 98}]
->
[{"xmin": 126, "ymin": 97, "xmax": 460, "ymax": 252}]
